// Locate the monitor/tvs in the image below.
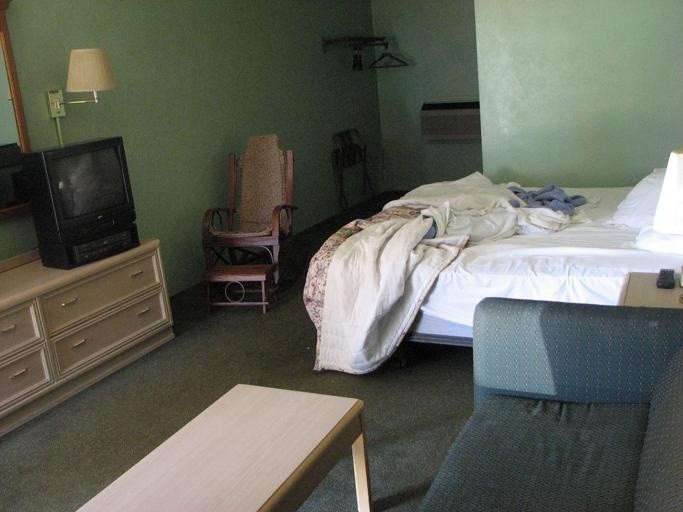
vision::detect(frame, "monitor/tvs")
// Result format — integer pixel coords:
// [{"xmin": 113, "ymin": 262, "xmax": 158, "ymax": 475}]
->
[
  {"xmin": 0, "ymin": 143, "xmax": 30, "ymax": 210},
  {"xmin": 20, "ymin": 136, "xmax": 140, "ymax": 269}
]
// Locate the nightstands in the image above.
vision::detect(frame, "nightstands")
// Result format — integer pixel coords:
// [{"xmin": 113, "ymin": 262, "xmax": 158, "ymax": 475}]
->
[{"xmin": 620, "ymin": 271, "xmax": 682, "ymax": 312}]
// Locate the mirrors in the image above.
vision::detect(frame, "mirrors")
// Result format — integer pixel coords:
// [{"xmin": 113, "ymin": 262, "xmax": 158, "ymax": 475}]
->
[{"xmin": 1, "ymin": 11, "xmax": 36, "ymax": 223}]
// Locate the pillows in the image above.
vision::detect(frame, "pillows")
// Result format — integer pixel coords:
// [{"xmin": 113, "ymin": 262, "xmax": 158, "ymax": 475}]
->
[{"xmin": 615, "ymin": 167, "xmax": 663, "ymax": 227}]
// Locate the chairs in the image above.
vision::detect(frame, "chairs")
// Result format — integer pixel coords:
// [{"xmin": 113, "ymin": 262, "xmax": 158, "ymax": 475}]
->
[
  {"xmin": 201, "ymin": 132, "xmax": 294, "ymax": 317},
  {"xmin": 332, "ymin": 128, "xmax": 376, "ymax": 213}
]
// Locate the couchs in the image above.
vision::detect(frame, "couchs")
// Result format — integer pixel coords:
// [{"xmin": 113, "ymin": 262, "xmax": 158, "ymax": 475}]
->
[{"xmin": 416, "ymin": 297, "xmax": 683, "ymax": 512}]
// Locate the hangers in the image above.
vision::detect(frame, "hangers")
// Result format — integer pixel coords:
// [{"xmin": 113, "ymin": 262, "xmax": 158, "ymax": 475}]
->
[{"xmin": 365, "ymin": 49, "xmax": 408, "ymax": 70}]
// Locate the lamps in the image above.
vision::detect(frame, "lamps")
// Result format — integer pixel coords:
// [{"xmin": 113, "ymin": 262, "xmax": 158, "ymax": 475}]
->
[
  {"xmin": 54, "ymin": 47, "xmax": 114, "ymax": 106},
  {"xmin": 653, "ymin": 148, "xmax": 683, "ymax": 236}
]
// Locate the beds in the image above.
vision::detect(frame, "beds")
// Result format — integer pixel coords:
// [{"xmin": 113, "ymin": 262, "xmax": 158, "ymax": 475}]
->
[{"xmin": 308, "ymin": 165, "xmax": 683, "ymax": 348}]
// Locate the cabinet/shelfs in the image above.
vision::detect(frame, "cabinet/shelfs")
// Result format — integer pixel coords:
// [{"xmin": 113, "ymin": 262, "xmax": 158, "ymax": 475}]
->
[
  {"xmin": 0, "ymin": 291, "xmax": 58, "ymax": 439},
  {"xmin": 31, "ymin": 238, "xmax": 176, "ymax": 399}
]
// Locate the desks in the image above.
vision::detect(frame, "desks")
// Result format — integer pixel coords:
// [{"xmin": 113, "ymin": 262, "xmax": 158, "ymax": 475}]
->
[{"xmin": 74, "ymin": 383, "xmax": 373, "ymax": 512}]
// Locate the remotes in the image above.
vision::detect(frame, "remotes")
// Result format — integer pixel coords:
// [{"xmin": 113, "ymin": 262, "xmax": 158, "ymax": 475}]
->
[{"xmin": 657, "ymin": 269, "xmax": 674, "ymax": 288}]
